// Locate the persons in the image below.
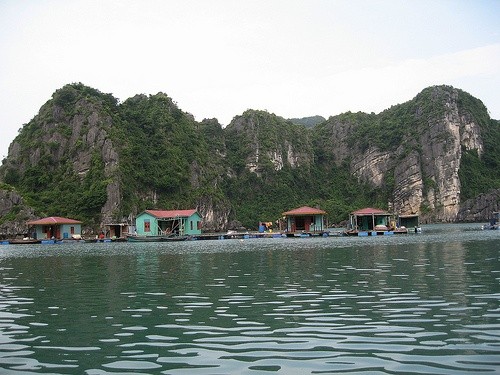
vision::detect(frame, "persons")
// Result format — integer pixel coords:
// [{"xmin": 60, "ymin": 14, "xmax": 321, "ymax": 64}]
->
[{"xmin": 259, "ymin": 223, "xmax": 265, "ymax": 238}]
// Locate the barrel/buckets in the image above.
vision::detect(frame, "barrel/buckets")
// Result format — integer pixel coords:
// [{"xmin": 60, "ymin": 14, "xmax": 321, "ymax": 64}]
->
[{"xmin": 112, "ymin": 236, "xmax": 116, "ymax": 241}]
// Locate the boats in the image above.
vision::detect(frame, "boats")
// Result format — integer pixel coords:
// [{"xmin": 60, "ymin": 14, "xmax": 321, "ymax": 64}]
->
[
  {"xmin": 122, "ymin": 231, "xmax": 177, "ymax": 242},
  {"xmin": 160, "ymin": 236, "xmax": 193, "ymax": 242}
]
